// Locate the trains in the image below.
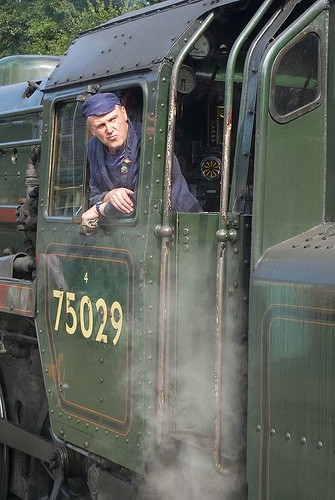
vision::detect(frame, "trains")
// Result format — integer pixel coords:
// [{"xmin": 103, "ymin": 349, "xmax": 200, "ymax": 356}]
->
[{"xmin": 2, "ymin": 0, "xmax": 335, "ymax": 500}]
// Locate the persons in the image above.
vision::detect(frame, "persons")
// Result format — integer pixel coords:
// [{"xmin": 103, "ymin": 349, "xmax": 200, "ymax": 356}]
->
[{"xmin": 82, "ymin": 93, "xmax": 206, "ymax": 235}]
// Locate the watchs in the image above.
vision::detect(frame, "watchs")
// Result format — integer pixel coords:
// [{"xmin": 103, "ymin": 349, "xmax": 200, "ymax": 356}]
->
[{"xmin": 97, "ymin": 202, "xmax": 105, "ymax": 216}]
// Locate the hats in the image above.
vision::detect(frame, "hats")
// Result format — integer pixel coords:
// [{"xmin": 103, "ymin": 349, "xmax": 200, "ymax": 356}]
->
[{"xmin": 82, "ymin": 93, "xmax": 121, "ymax": 119}]
